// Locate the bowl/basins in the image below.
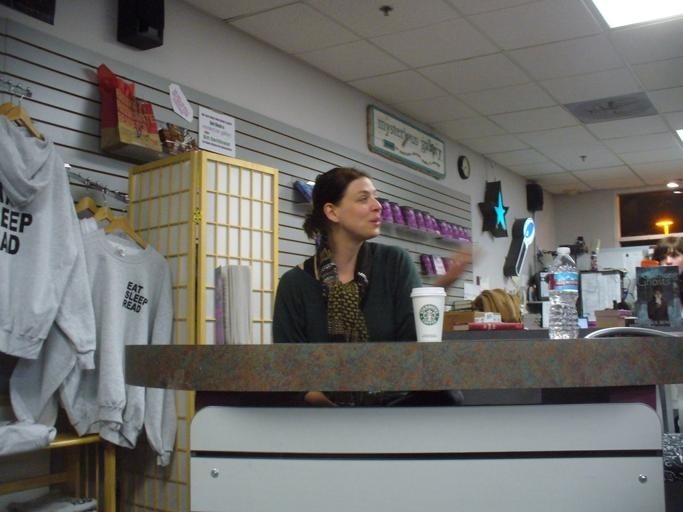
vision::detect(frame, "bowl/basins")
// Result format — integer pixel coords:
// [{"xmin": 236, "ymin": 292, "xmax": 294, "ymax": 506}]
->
[{"xmin": 642, "ymin": 260, "xmax": 659, "ymax": 266}]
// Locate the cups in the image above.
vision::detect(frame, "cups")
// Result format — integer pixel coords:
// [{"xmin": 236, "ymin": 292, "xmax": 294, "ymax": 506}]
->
[
  {"xmin": 522, "ymin": 313, "xmax": 542, "ymax": 329},
  {"xmin": 410, "ymin": 287, "xmax": 447, "ymax": 342}
]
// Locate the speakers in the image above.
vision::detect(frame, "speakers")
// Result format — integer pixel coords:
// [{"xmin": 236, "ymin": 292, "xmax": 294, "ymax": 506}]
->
[
  {"xmin": 526, "ymin": 183, "xmax": 544, "ymax": 211},
  {"xmin": 115, "ymin": 0, "xmax": 166, "ymax": 51}
]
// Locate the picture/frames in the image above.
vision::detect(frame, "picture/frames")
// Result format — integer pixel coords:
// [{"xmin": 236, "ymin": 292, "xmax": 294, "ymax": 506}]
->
[{"xmin": 368, "ymin": 104, "xmax": 447, "ymax": 178}]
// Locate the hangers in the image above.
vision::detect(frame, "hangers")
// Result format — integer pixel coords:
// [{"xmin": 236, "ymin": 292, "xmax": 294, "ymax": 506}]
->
[
  {"xmin": 1, "ymin": 80, "xmax": 44, "ymax": 142},
  {"xmin": 75, "ymin": 177, "xmax": 148, "ymax": 249}
]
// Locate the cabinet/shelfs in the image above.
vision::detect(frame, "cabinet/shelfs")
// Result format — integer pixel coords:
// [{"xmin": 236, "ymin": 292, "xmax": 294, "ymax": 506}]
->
[
  {"xmin": 1, "ymin": 434, "xmax": 119, "ymax": 512},
  {"xmin": 292, "ymin": 200, "xmax": 471, "ymax": 278}
]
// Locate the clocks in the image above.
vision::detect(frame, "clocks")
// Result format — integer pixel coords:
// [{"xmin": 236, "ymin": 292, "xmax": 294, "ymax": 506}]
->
[{"xmin": 458, "ymin": 155, "xmax": 470, "ymax": 180}]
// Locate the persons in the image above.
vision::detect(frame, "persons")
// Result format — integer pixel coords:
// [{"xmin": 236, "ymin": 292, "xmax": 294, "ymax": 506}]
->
[
  {"xmin": 272, "ymin": 168, "xmax": 466, "ymax": 406},
  {"xmin": 652, "ymin": 237, "xmax": 682, "ymax": 304},
  {"xmin": 648, "ymin": 285, "xmax": 670, "ymax": 327}
]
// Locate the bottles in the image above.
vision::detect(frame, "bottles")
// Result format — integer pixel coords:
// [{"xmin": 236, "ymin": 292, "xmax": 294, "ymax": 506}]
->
[
  {"xmin": 576, "ymin": 236, "xmax": 585, "ymax": 252},
  {"xmin": 548, "ymin": 246, "xmax": 578, "ymax": 340},
  {"xmin": 590, "ymin": 250, "xmax": 599, "ymax": 271}
]
